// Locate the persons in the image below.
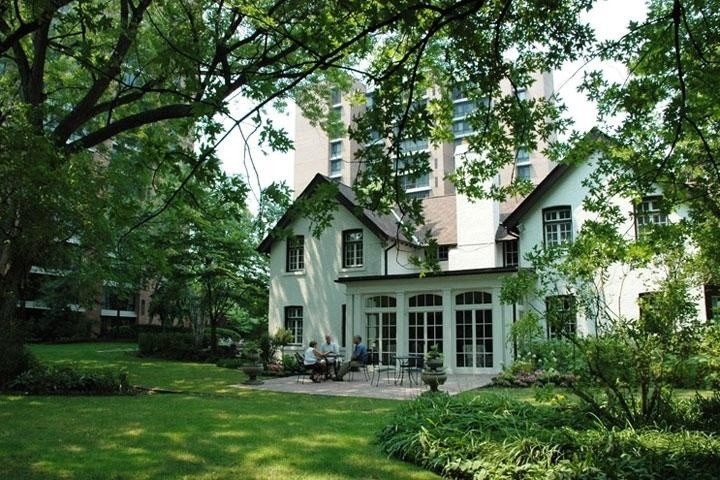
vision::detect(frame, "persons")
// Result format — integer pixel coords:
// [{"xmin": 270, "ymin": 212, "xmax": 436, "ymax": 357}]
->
[
  {"xmin": 304, "ymin": 334, "xmax": 339, "ymax": 383},
  {"xmin": 332, "ymin": 335, "xmax": 368, "ymax": 381}
]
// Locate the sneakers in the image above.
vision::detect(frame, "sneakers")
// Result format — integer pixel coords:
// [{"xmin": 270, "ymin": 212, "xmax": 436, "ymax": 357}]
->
[
  {"xmin": 332, "ymin": 376, "xmax": 343, "ymax": 381},
  {"xmin": 310, "ymin": 376, "xmax": 321, "ymax": 383}
]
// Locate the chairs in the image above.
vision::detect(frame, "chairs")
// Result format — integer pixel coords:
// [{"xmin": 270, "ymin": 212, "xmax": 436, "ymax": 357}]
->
[{"xmin": 294, "ymin": 346, "xmax": 423, "ymax": 388}]
